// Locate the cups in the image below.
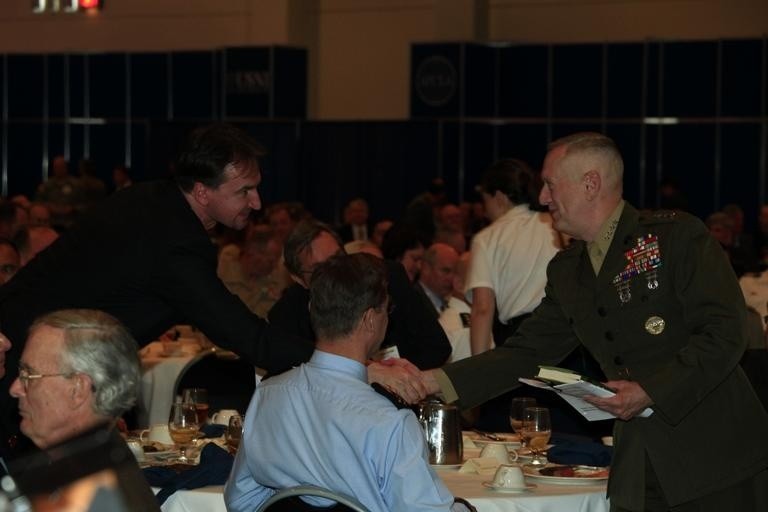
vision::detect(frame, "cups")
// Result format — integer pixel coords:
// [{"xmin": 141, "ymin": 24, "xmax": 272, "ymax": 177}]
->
[
  {"xmin": 211, "ymin": 410, "xmax": 242, "ymax": 426},
  {"xmin": 140, "ymin": 424, "xmax": 174, "ymax": 445},
  {"xmin": 478, "ymin": 443, "xmax": 518, "ymax": 465},
  {"xmin": 184, "ymin": 389, "xmax": 208, "ymax": 425},
  {"xmin": 490, "ymin": 464, "xmax": 526, "ymax": 488}
]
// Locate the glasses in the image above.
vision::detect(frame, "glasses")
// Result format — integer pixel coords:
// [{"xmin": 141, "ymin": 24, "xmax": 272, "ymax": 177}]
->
[
  {"xmin": 16, "ymin": 360, "xmax": 99, "ymax": 394},
  {"xmin": 427, "ymin": 183, "xmax": 448, "ymax": 193},
  {"xmin": 296, "ymin": 267, "xmax": 315, "ymax": 277},
  {"xmin": 370, "ymin": 299, "xmax": 399, "ymax": 317}
]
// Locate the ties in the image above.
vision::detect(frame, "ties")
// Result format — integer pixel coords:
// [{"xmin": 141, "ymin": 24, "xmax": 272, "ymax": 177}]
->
[{"xmin": 357, "ymin": 224, "xmax": 366, "ymax": 240}]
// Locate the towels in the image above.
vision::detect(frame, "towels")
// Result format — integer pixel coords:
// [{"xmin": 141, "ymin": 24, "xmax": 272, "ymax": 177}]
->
[
  {"xmin": 199, "ymin": 424, "xmax": 226, "ymax": 438},
  {"xmin": 548, "ymin": 439, "xmax": 609, "ymax": 466},
  {"xmin": 548, "ymin": 431, "xmax": 603, "ymax": 444},
  {"xmin": 142, "ymin": 442, "xmax": 234, "ymax": 506}
]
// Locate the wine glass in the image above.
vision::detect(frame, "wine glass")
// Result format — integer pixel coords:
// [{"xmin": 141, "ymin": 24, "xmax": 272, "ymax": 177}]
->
[
  {"xmin": 510, "ymin": 398, "xmax": 536, "ymax": 455},
  {"xmin": 229, "ymin": 414, "xmax": 245, "ymax": 439},
  {"xmin": 523, "ymin": 407, "xmax": 551, "ymax": 465},
  {"xmin": 168, "ymin": 402, "xmax": 196, "ymax": 462}
]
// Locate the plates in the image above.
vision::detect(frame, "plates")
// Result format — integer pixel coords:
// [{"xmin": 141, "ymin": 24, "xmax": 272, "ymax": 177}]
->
[
  {"xmin": 522, "ymin": 464, "xmax": 610, "ymax": 486},
  {"xmin": 481, "ymin": 481, "xmax": 538, "ymax": 494},
  {"xmin": 145, "ymin": 452, "xmax": 176, "ymax": 457}
]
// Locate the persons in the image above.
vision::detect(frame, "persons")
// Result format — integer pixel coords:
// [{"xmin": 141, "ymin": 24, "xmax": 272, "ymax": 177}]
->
[
  {"xmin": 400, "ymin": 178, "xmax": 446, "ymax": 247},
  {"xmin": 382, "ymin": 224, "xmax": 425, "ymax": 294},
  {"xmin": 215, "ymin": 221, "xmax": 292, "ymax": 324},
  {"xmin": 0, "ymin": 309, "xmax": 162, "ymax": 512},
  {"xmin": 434, "ymin": 205, "xmax": 466, "ymax": 256},
  {"xmin": 704, "ymin": 212, "xmax": 733, "ymax": 268},
  {"xmin": 658, "ymin": 181, "xmax": 675, "ymax": 208},
  {"xmin": 380, "ymin": 132, "xmax": 768, "ymax": 508},
  {"xmin": 32, "ymin": 154, "xmax": 107, "ymax": 221},
  {"xmin": 223, "ymin": 251, "xmax": 455, "ymax": 512},
  {"xmin": 0, "ymin": 123, "xmax": 427, "ymax": 405},
  {"xmin": 720, "ymin": 204, "xmax": 755, "ymax": 272},
  {"xmin": 438, "ymin": 249, "xmax": 496, "ymax": 368},
  {"xmin": 0, "ymin": 236, "xmax": 22, "ymax": 286},
  {"xmin": 363, "ymin": 216, "xmax": 394, "ymax": 260},
  {"xmin": 9, "ymin": 222, "xmax": 58, "ymax": 267},
  {"xmin": 0, "ymin": 332, "xmax": 12, "ymax": 379},
  {"xmin": 758, "ymin": 204, "xmax": 768, "ymax": 270},
  {"xmin": 109, "ymin": 163, "xmax": 136, "ymax": 193},
  {"xmin": 385, "ymin": 242, "xmax": 460, "ymax": 370},
  {"xmin": 466, "ymin": 204, "xmax": 493, "ymax": 251},
  {"xmin": 338, "ymin": 195, "xmax": 374, "ymax": 244},
  {"xmin": 0, "ymin": 192, "xmax": 52, "ymax": 236},
  {"xmin": 260, "ymin": 220, "xmax": 346, "ymax": 380},
  {"xmin": 464, "ymin": 159, "xmax": 573, "ymax": 356},
  {"xmin": 263, "ymin": 202, "xmax": 293, "ymax": 241}
]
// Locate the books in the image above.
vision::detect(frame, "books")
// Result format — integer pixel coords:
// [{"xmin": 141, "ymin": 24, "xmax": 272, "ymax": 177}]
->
[{"xmin": 517, "ymin": 364, "xmax": 653, "ymax": 423}]
